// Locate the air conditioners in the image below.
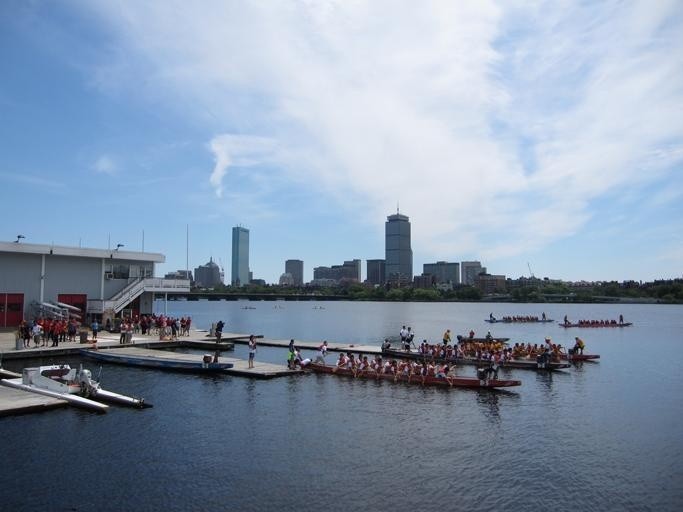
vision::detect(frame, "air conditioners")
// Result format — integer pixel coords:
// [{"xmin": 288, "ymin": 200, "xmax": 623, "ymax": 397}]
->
[{"xmin": 105, "ymin": 273, "xmax": 115, "ymax": 280}]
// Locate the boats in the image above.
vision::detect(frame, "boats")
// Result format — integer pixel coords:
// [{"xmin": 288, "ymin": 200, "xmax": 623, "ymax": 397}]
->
[
  {"xmin": 31, "ymin": 365, "xmax": 100, "ymax": 394},
  {"xmin": 485, "ymin": 319, "xmax": 632, "ymax": 327},
  {"xmin": 79, "ymin": 349, "xmax": 233, "ymax": 370},
  {"xmin": 307, "ymin": 337, "xmax": 600, "ymax": 387}
]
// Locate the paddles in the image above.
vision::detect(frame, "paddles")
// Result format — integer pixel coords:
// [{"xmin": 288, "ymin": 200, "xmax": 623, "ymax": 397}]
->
[
  {"xmin": 331, "ymin": 363, "xmax": 345, "ymax": 372},
  {"xmin": 353, "ymin": 365, "xmax": 356, "ymax": 378},
  {"xmin": 448, "ymin": 370, "xmax": 453, "ymax": 387},
  {"xmin": 375, "ymin": 366, "xmax": 383, "ymax": 379},
  {"xmin": 406, "ymin": 367, "xmax": 412, "ymax": 384},
  {"xmin": 393, "ymin": 369, "xmax": 397, "ymax": 383},
  {"xmin": 421, "ymin": 374, "xmax": 425, "ymax": 386}
]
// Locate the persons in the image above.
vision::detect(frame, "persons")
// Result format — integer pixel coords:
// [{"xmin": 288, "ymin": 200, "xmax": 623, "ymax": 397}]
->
[
  {"xmin": 17, "ymin": 318, "xmax": 78, "ymax": 348},
  {"xmin": 397, "ymin": 324, "xmax": 563, "ymax": 373},
  {"xmin": 214, "ymin": 321, "xmax": 225, "ymax": 344},
  {"xmin": 619, "ymin": 312, "xmax": 624, "ymax": 324},
  {"xmin": 332, "ymin": 351, "xmax": 457, "ymax": 389},
  {"xmin": 502, "ymin": 315, "xmax": 538, "ymax": 322},
  {"xmin": 119, "ymin": 313, "xmax": 191, "ymax": 344},
  {"xmin": 89, "ymin": 319, "xmax": 98, "ymax": 340},
  {"xmin": 247, "ymin": 334, "xmax": 256, "ymax": 368},
  {"xmin": 381, "ymin": 338, "xmax": 391, "ymax": 353},
  {"xmin": 288, "ymin": 339, "xmax": 313, "ymax": 371},
  {"xmin": 490, "ymin": 313, "xmax": 496, "ymax": 320},
  {"xmin": 563, "ymin": 314, "xmax": 570, "ymax": 324},
  {"xmin": 313, "ymin": 340, "xmax": 328, "ymax": 366},
  {"xmin": 541, "ymin": 312, "xmax": 547, "ymax": 320},
  {"xmin": 579, "ymin": 319, "xmax": 616, "ymax": 325},
  {"xmin": 574, "ymin": 336, "xmax": 585, "ymax": 355}
]
